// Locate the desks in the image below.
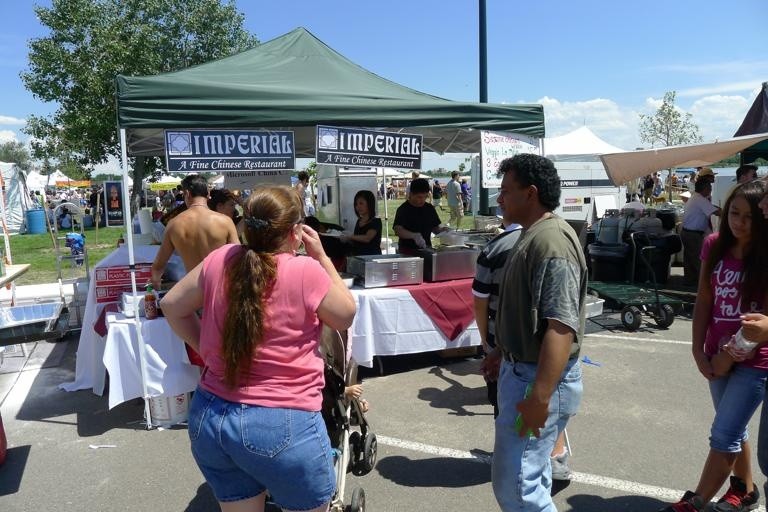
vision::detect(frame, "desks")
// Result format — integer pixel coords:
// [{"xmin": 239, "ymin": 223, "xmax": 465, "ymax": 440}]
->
[
  {"xmin": 0, "ymin": 259, "xmax": 35, "ymax": 366},
  {"xmin": 92, "ymin": 283, "xmax": 361, "ymax": 434},
  {"xmin": 344, "ymin": 279, "xmax": 481, "ymax": 383}
]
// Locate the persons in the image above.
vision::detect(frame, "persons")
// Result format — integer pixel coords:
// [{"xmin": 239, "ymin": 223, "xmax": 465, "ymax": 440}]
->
[
  {"xmin": 667, "ymin": 180, "xmax": 767, "ymax": 512},
  {"xmin": 333, "ymin": 189, "xmax": 384, "ymax": 256},
  {"xmin": 472, "ymin": 193, "xmax": 576, "ymax": 487},
  {"xmin": 625, "ymin": 165, "xmax": 759, "ymax": 207},
  {"xmin": 147, "ymin": 174, "xmax": 241, "ymax": 316},
  {"xmin": 491, "ymin": 155, "xmax": 592, "ymax": 511},
  {"xmin": 680, "ymin": 177, "xmax": 722, "ymax": 285},
  {"xmin": 392, "ymin": 179, "xmax": 456, "ymax": 281},
  {"xmin": 152, "ymin": 170, "xmax": 317, "ymax": 240},
  {"xmin": 739, "ymin": 194, "xmax": 767, "ymax": 509},
  {"xmin": 405, "ymin": 170, "xmax": 473, "ymax": 215},
  {"xmin": 30, "ymin": 186, "xmax": 103, "ymax": 230},
  {"xmin": 444, "ymin": 170, "xmax": 464, "ymax": 232},
  {"xmin": 303, "ymin": 215, "xmax": 347, "ymax": 274},
  {"xmin": 158, "ymin": 184, "xmax": 359, "ymax": 512}
]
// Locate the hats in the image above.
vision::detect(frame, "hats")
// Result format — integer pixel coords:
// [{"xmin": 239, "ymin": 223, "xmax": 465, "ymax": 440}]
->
[{"xmin": 697, "ymin": 167, "xmax": 716, "ymax": 177}]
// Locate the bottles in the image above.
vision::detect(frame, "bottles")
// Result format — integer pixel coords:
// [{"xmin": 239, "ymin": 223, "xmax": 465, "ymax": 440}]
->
[
  {"xmin": 145, "ymin": 286, "xmax": 157, "ymax": 318},
  {"xmin": 515, "ymin": 383, "xmax": 543, "ymax": 437}
]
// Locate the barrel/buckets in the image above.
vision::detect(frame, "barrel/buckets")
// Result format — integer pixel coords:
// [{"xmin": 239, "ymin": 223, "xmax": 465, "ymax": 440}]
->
[
  {"xmin": 26, "ymin": 209, "xmax": 47, "ymax": 234},
  {"xmin": 150, "ymin": 392, "xmax": 192, "ymax": 428}
]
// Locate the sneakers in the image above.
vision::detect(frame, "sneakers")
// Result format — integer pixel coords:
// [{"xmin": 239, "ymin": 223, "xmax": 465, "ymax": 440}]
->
[
  {"xmin": 550, "ymin": 444, "xmax": 571, "ymax": 483},
  {"xmin": 655, "ymin": 490, "xmax": 712, "ymax": 512},
  {"xmin": 712, "ymin": 476, "xmax": 760, "ymax": 512}
]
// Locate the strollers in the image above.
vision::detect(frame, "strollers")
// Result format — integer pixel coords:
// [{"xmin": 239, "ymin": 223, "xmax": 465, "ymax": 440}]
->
[{"xmin": 263, "ymin": 315, "xmax": 381, "ymax": 512}]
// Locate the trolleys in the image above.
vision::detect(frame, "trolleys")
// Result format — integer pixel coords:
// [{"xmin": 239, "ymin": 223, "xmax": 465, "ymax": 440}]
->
[
  {"xmin": 585, "ymin": 229, "xmax": 688, "ymax": 333},
  {"xmin": 49, "ymin": 201, "xmax": 94, "ymax": 336}
]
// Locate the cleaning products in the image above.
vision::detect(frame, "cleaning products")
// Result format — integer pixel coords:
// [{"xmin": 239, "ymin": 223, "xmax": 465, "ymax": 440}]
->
[{"xmin": 144, "ymin": 277, "xmax": 159, "ymax": 319}]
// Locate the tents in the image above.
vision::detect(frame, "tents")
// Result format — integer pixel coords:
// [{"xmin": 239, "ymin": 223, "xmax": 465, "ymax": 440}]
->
[{"xmin": 0, "ymin": 160, "xmax": 33, "ymax": 237}]
